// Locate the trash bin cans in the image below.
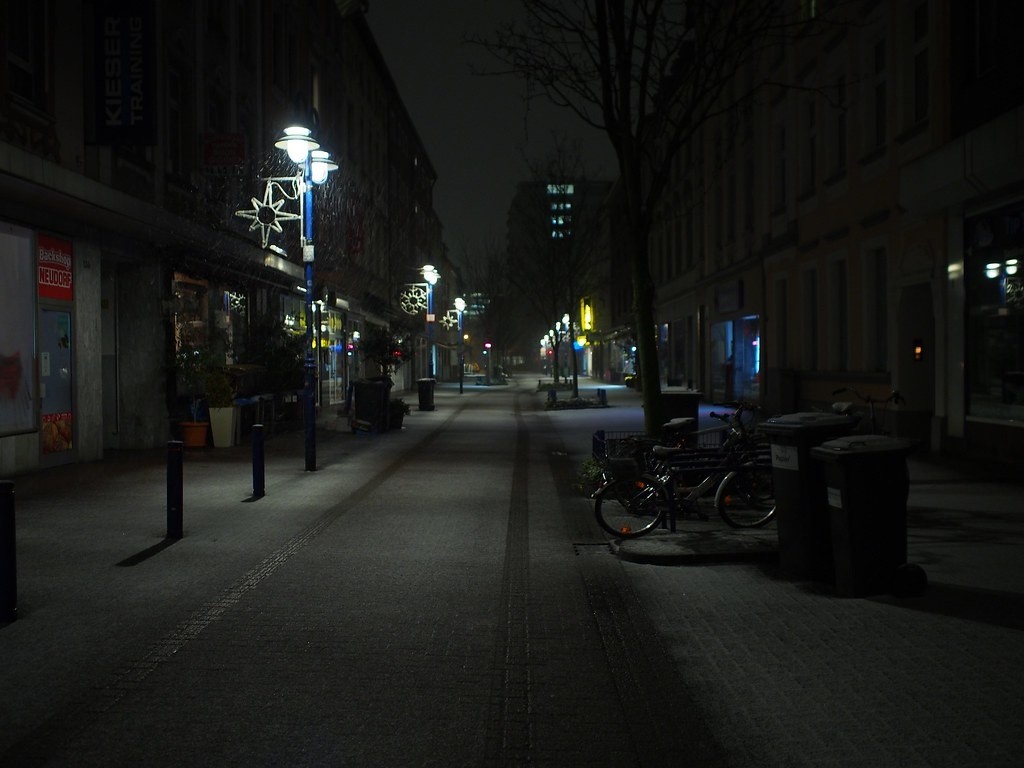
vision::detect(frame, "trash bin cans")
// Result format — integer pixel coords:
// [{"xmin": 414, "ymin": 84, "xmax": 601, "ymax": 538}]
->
[
  {"xmin": 351, "ymin": 374, "xmax": 394, "ymax": 428},
  {"xmin": 415, "ymin": 377, "xmax": 438, "ymax": 412},
  {"xmin": 752, "ymin": 404, "xmax": 913, "ymax": 595},
  {"xmin": 644, "ymin": 384, "xmax": 704, "ymax": 456}
]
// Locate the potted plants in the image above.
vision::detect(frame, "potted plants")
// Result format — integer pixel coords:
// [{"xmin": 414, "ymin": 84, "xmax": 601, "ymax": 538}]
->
[
  {"xmin": 390, "ymin": 397, "xmax": 412, "ymax": 430},
  {"xmin": 577, "ymin": 456, "xmax": 612, "ymax": 497},
  {"xmin": 176, "ymin": 308, "xmax": 313, "ymax": 448}
]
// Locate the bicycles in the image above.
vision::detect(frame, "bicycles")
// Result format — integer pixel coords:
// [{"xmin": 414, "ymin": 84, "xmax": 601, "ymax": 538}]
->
[
  {"xmin": 714, "ymin": 387, "xmax": 906, "ymax": 528},
  {"xmin": 590, "ymin": 403, "xmax": 776, "ymax": 540}
]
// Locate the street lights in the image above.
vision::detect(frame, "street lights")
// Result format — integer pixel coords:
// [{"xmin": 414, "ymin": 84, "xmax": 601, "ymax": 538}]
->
[
  {"xmin": 985, "ymin": 259, "xmax": 1017, "ymax": 306},
  {"xmin": 420, "ymin": 264, "xmax": 441, "ymax": 377},
  {"xmin": 454, "ymin": 298, "xmax": 465, "ymax": 394},
  {"xmin": 274, "ymin": 124, "xmax": 339, "ymax": 472}
]
